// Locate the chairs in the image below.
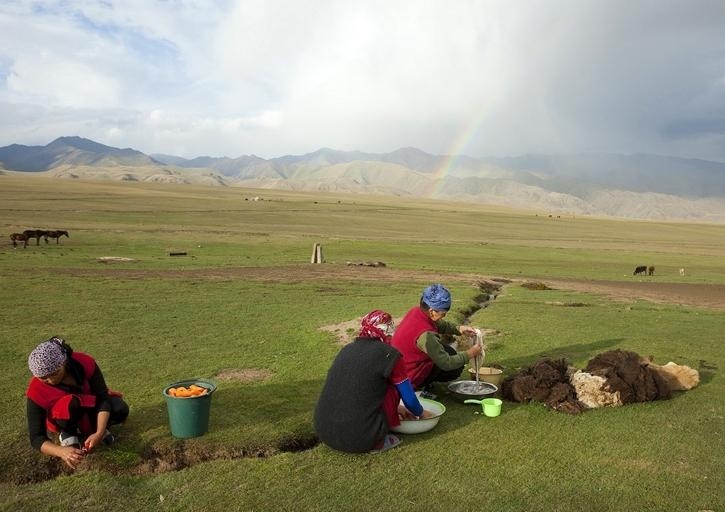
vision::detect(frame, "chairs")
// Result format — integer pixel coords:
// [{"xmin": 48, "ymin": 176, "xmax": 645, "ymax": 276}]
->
[
  {"xmin": 420, "ymin": 390, "xmax": 438, "ymax": 399},
  {"xmin": 102, "ymin": 428, "xmax": 115, "ymax": 446},
  {"xmin": 58, "ymin": 431, "xmax": 81, "ymax": 451}
]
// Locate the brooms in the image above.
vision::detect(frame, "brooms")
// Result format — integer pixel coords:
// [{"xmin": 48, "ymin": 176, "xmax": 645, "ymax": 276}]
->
[{"xmin": 168, "ymin": 384, "xmax": 207, "ymax": 398}]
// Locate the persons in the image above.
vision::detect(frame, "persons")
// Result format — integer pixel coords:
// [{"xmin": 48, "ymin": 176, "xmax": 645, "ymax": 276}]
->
[
  {"xmin": 23, "ymin": 334, "xmax": 130, "ymax": 471},
  {"xmin": 390, "ymin": 282, "xmax": 482, "ymax": 401},
  {"xmin": 311, "ymin": 308, "xmax": 433, "ymax": 454}
]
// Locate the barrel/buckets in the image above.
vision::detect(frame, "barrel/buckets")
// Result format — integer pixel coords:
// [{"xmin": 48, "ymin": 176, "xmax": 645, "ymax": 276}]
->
[{"xmin": 369, "ymin": 434, "xmax": 404, "ymax": 455}]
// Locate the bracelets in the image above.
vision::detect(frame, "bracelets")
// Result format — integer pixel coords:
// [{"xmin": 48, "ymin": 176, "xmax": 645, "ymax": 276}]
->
[
  {"xmin": 447, "ymin": 366, "xmax": 503, "ymax": 403},
  {"xmin": 391, "ymin": 396, "xmax": 446, "ymax": 434}
]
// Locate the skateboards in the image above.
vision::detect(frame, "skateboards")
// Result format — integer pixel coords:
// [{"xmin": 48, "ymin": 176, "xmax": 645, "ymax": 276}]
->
[
  {"xmin": 679, "ymin": 267, "xmax": 685, "ymax": 276},
  {"xmin": 633, "ymin": 266, "xmax": 647, "ymax": 276},
  {"xmin": 648, "ymin": 266, "xmax": 655, "ymax": 276}
]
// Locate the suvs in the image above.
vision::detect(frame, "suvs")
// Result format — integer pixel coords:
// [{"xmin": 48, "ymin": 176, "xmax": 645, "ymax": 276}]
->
[{"xmin": 162, "ymin": 380, "xmax": 218, "ymax": 440}]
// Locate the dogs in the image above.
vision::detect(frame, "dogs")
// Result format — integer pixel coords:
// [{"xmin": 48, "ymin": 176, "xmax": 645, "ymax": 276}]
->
[
  {"xmin": 9, "ymin": 233, "xmax": 30, "ymax": 249},
  {"xmin": 44, "ymin": 230, "xmax": 69, "ymax": 244},
  {"xmin": 23, "ymin": 230, "xmax": 45, "ymax": 246}
]
